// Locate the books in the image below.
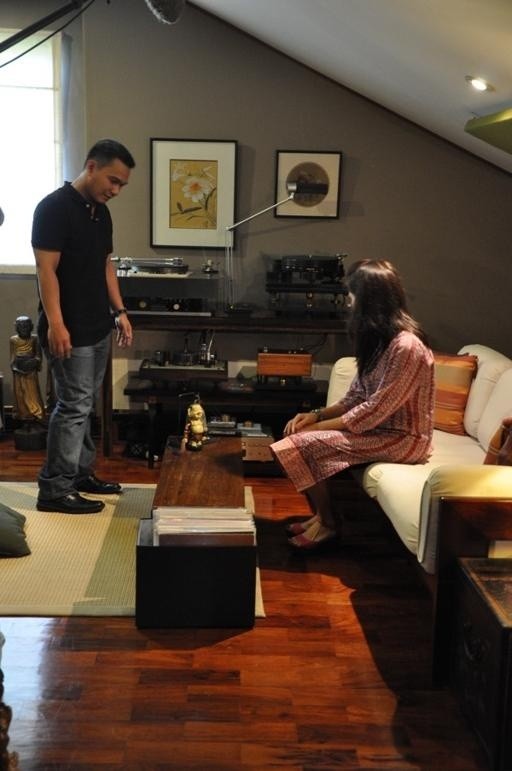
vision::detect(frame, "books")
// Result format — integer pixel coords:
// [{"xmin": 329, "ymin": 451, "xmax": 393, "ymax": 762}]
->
[{"xmin": 151, "ymin": 507, "xmax": 258, "ymax": 548}]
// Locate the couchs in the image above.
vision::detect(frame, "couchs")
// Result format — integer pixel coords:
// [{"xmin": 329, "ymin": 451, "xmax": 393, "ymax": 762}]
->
[{"xmin": 327, "ymin": 343, "xmax": 512, "ymax": 557}]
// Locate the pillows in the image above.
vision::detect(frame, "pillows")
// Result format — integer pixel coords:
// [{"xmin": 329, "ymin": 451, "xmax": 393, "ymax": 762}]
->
[{"xmin": 428, "ymin": 351, "xmax": 478, "ymax": 436}]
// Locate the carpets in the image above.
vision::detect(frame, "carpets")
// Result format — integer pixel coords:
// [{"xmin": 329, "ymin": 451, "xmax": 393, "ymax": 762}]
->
[{"xmin": 0, "ymin": 480, "xmax": 267, "ymax": 620}]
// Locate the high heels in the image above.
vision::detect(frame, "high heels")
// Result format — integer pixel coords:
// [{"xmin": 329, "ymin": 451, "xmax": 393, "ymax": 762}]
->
[{"xmin": 285, "ymin": 520, "xmax": 338, "ymax": 557}]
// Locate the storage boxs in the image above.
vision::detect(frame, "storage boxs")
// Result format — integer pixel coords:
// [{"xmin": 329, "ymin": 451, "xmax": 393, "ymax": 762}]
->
[{"xmin": 135, "ymin": 518, "xmax": 258, "ymax": 630}]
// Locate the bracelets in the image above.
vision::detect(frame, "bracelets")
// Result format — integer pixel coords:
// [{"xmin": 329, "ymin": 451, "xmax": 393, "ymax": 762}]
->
[
  {"xmin": 312, "ymin": 408, "xmax": 323, "ymax": 422},
  {"xmin": 113, "ymin": 308, "xmax": 127, "ymax": 320}
]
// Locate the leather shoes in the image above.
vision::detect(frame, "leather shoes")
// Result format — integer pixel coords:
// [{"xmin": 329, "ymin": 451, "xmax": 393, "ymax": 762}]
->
[
  {"xmin": 37, "ymin": 494, "xmax": 105, "ymax": 514},
  {"xmin": 77, "ymin": 474, "xmax": 121, "ymax": 494}
]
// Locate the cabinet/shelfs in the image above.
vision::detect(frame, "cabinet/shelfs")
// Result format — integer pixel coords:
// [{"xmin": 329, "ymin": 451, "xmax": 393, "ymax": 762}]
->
[
  {"xmin": 99, "ymin": 295, "xmax": 359, "ymax": 455},
  {"xmin": 447, "ymin": 558, "xmax": 512, "ymax": 771}
]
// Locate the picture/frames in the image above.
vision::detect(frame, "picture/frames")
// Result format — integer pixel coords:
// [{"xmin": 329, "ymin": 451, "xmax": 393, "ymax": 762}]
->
[
  {"xmin": 273, "ymin": 148, "xmax": 342, "ymax": 219},
  {"xmin": 148, "ymin": 138, "xmax": 239, "ymax": 251}
]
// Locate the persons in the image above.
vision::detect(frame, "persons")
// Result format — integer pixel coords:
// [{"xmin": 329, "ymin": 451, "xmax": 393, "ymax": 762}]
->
[
  {"xmin": 267, "ymin": 256, "xmax": 437, "ymax": 557},
  {"xmin": 31, "ymin": 137, "xmax": 135, "ymax": 515},
  {"xmin": 181, "ymin": 398, "xmax": 211, "ymax": 452},
  {"xmin": 9, "ymin": 314, "xmax": 48, "ymax": 428}
]
